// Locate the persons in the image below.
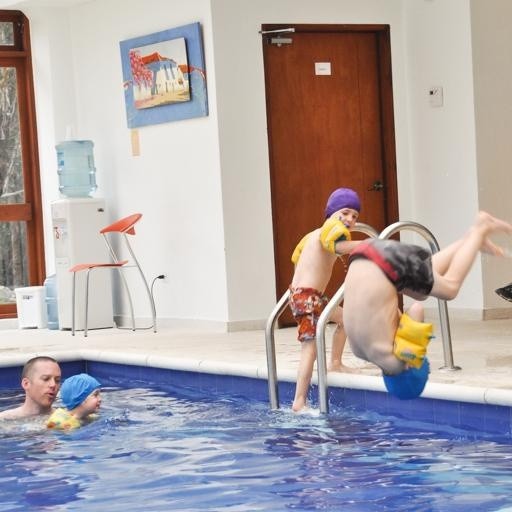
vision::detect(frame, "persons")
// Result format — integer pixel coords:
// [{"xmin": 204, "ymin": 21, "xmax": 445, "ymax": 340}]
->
[
  {"xmin": 45, "ymin": 371, "xmax": 106, "ymax": 430},
  {"xmin": 291, "ymin": 187, "xmax": 361, "ymax": 414},
  {"xmin": 342, "ymin": 211, "xmax": 512, "ymax": 399},
  {"xmin": 1, "ymin": 355, "xmax": 62, "ymax": 423}
]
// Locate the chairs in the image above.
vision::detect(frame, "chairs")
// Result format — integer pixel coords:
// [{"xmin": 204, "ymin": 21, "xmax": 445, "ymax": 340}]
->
[{"xmin": 69, "ymin": 213, "xmax": 158, "ymax": 338}]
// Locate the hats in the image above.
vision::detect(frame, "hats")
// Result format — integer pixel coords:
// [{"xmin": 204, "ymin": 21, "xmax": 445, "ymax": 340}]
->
[
  {"xmin": 61, "ymin": 373, "xmax": 101, "ymax": 410},
  {"xmin": 326, "ymin": 188, "xmax": 360, "ymax": 218},
  {"xmin": 383, "ymin": 358, "xmax": 429, "ymax": 400}
]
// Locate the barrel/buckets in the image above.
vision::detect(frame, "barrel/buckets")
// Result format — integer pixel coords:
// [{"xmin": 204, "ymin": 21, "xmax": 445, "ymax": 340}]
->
[
  {"xmin": 56, "ymin": 140, "xmax": 98, "ymax": 196},
  {"xmin": 44, "ymin": 273, "xmax": 60, "ymax": 330},
  {"xmin": 381, "ymin": 355, "xmax": 431, "ymax": 398}
]
